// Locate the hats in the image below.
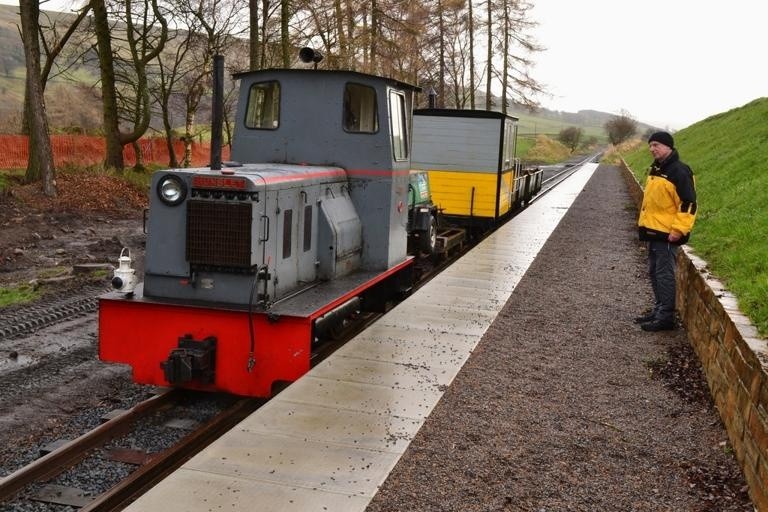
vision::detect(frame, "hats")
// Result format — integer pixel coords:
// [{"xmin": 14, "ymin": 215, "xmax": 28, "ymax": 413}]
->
[{"xmin": 649, "ymin": 132, "xmax": 673, "ymax": 149}]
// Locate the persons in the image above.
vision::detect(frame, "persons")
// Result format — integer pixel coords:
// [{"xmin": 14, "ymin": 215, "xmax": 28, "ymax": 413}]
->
[{"xmin": 633, "ymin": 130, "xmax": 700, "ymax": 332}]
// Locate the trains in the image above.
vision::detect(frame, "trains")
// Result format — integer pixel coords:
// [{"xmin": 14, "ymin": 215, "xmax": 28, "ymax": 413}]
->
[{"xmin": 92, "ymin": 42, "xmax": 547, "ymax": 406}]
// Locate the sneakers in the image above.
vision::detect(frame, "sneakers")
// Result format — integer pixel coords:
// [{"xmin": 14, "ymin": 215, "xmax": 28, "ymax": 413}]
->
[
  {"xmin": 641, "ymin": 321, "xmax": 672, "ymax": 331},
  {"xmin": 639, "ymin": 314, "xmax": 654, "ymax": 322}
]
